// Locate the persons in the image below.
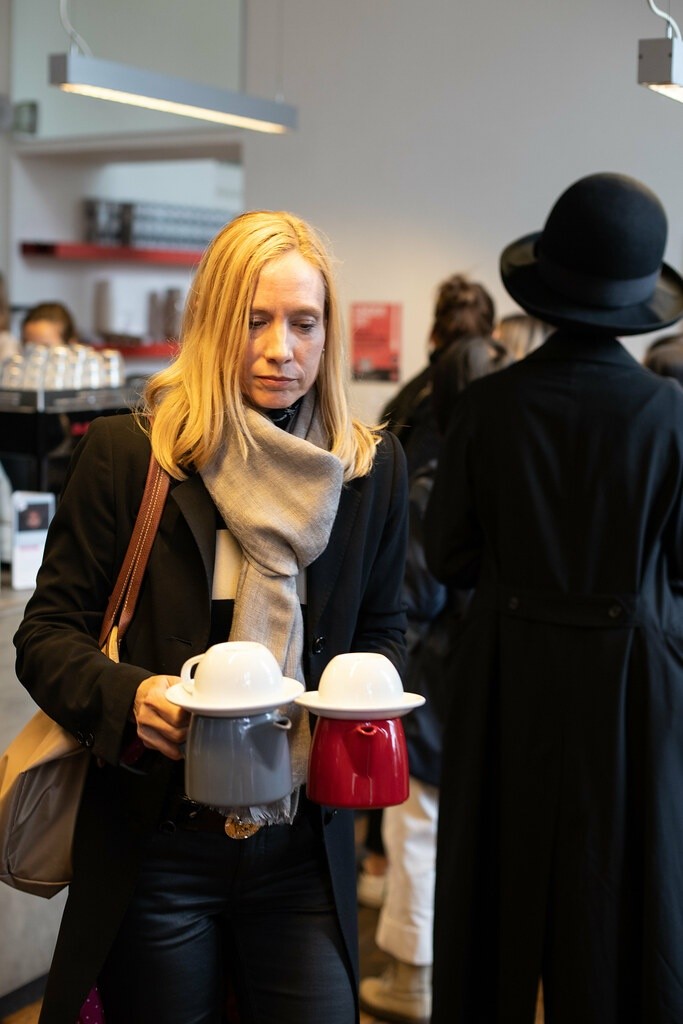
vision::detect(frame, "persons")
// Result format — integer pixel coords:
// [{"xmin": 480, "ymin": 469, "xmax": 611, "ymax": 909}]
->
[
  {"xmin": 360, "ymin": 275, "xmax": 683, "ymax": 1024},
  {"xmin": 422, "ymin": 173, "xmax": 683, "ymax": 1024},
  {"xmin": 0, "ymin": 302, "xmax": 130, "ymax": 508},
  {"xmin": 13, "ymin": 211, "xmax": 411, "ymax": 1024}
]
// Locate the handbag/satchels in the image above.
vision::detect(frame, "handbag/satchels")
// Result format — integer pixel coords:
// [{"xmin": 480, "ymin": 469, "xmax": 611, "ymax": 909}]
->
[{"xmin": 1, "ymin": 625, "xmax": 118, "ymax": 900}]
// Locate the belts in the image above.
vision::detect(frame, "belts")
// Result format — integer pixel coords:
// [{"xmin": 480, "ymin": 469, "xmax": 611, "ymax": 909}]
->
[{"xmin": 164, "ymin": 798, "xmax": 306, "ymax": 840}]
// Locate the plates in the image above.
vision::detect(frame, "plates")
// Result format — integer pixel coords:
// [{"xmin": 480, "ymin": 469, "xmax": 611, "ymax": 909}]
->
[
  {"xmin": 296, "ymin": 690, "xmax": 426, "ymax": 720},
  {"xmin": 165, "ymin": 677, "xmax": 304, "ymax": 717}
]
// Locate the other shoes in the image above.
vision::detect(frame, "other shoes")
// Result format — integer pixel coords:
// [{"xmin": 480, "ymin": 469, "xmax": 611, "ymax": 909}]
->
[
  {"xmin": 357, "ymin": 859, "xmax": 386, "ymax": 909},
  {"xmin": 359, "ymin": 957, "xmax": 432, "ymax": 1024}
]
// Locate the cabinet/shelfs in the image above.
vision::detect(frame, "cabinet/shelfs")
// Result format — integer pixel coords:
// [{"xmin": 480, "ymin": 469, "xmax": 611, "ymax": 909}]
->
[{"xmin": 21, "ymin": 242, "xmax": 207, "ymax": 359}]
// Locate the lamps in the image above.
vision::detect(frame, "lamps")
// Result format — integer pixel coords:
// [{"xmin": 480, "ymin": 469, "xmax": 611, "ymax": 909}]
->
[
  {"xmin": 638, "ymin": 0, "xmax": 682, "ymax": 103},
  {"xmin": 43, "ymin": 0, "xmax": 296, "ymax": 135}
]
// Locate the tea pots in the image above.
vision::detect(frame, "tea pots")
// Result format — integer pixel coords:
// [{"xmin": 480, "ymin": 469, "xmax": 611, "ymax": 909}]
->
[
  {"xmin": 304, "ymin": 715, "xmax": 411, "ymax": 808},
  {"xmin": 174, "ymin": 710, "xmax": 294, "ymax": 806}
]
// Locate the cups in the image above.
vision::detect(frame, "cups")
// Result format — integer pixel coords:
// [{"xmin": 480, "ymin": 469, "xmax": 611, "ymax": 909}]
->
[
  {"xmin": 317, "ymin": 652, "xmax": 405, "ymax": 710},
  {"xmin": 181, "ymin": 640, "xmax": 286, "ymax": 702}
]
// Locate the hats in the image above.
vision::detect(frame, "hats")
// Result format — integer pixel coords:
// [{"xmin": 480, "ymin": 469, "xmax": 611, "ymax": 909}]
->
[{"xmin": 498, "ymin": 172, "xmax": 683, "ymax": 336}]
